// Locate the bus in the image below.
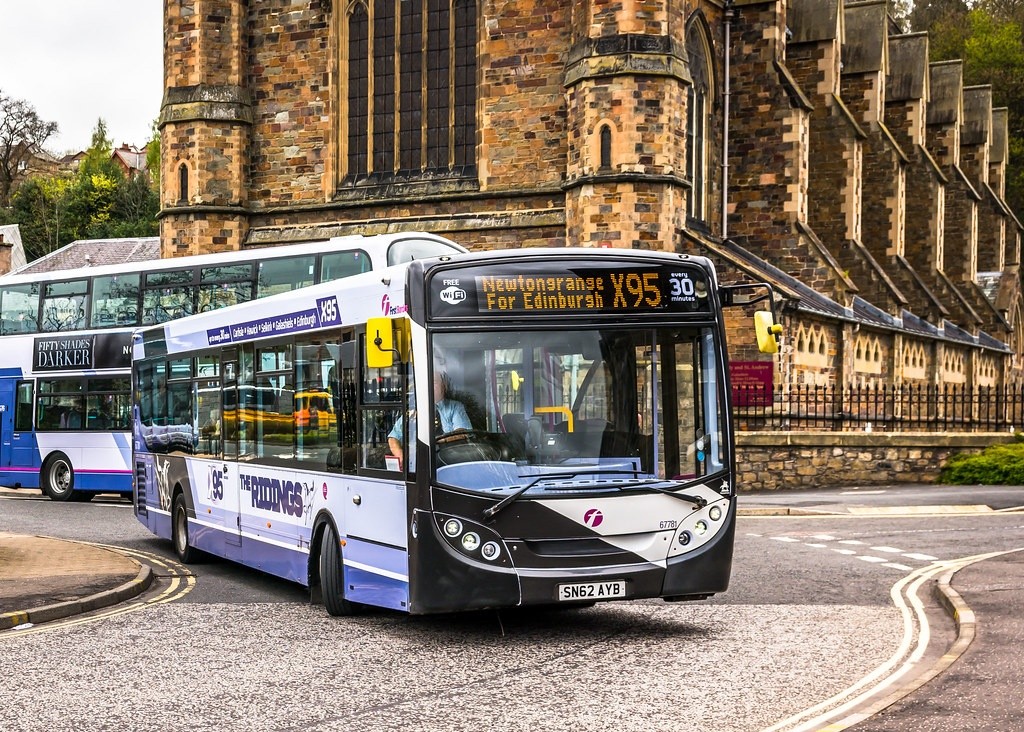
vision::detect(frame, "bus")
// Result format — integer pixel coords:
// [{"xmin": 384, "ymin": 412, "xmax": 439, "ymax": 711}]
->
[
  {"xmin": 134, "ymin": 249, "xmax": 785, "ymax": 618},
  {"xmin": 0, "ymin": 231, "xmax": 522, "ymax": 503}
]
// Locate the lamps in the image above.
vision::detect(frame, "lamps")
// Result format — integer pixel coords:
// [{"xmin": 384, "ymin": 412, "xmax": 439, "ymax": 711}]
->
[
  {"xmin": 82, "ymin": 255, "xmax": 93, "ymax": 268},
  {"xmin": 722, "ymin": 5, "xmax": 741, "ymax": 24},
  {"xmin": 786, "ymin": 296, "xmax": 801, "ymax": 314}
]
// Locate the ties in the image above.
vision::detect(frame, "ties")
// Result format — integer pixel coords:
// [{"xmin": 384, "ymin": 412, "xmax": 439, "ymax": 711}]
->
[{"xmin": 435, "ymin": 410, "xmax": 444, "ymax": 435}]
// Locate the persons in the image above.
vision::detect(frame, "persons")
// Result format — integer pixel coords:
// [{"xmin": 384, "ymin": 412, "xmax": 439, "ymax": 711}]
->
[{"xmin": 387, "ymin": 374, "xmax": 473, "ymax": 470}]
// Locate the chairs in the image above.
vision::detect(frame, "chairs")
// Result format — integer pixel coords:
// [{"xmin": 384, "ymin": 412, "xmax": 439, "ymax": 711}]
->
[
  {"xmin": 501, "ymin": 412, "xmax": 549, "ymax": 438},
  {"xmin": 0, "ymin": 297, "xmax": 229, "ymax": 335},
  {"xmin": 140, "ymin": 376, "xmax": 276, "ymax": 420},
  {"xmin": 58, "ymin": 412, "xmax": 83, "ymax": 429}
]
